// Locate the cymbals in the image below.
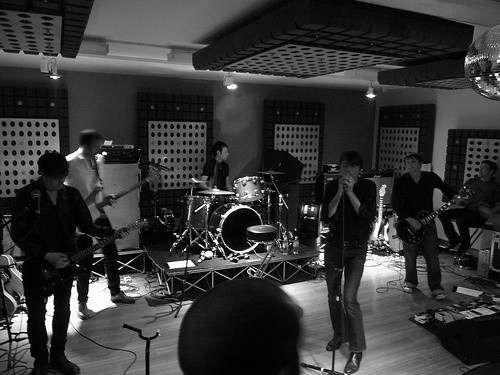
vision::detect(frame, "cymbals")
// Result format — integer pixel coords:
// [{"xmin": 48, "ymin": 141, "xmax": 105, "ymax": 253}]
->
[
  {"xmin": 197, "ymin": 188, "xmax": 235, "ymax": 195},
  {"xmin": 256, "ymin": 171, "xmax": 285, "ymax": 175},
  {"xmin": 187, "ymin": 180, "xmax": 212, "ymax": 183}
]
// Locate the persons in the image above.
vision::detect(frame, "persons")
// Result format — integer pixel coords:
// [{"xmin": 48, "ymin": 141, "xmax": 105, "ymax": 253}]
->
[
  {"xmin": 319, "ymin": 150, "xmax": 377, "ymax": 375},
  {"xmin": 63, "ymin": 129, "xmax": 135, "ymax": 320},
  {"xmin": 10, "ymin": 149, "xmax": 129, "ymax": 375},
  {"xmin": 438, "ymin": 160, "xmax": 500, "ymax": 253},
  {"xmin": 391, "ymin": 152, "xmax": 475, "ymax": 300},
  {"xmin": 199, "ymin": 141, "xmax": 232, "ymax": 191},
  {"xmin": 177, "ymin": 278, "xmax": 303, "ymax": 375}
]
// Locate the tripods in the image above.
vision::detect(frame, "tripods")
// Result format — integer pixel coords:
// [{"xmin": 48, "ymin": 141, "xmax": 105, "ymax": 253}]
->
[
  {"xmin": 0, "ymin": 208, "xmax": 33, "ymax": 345},
  {"xmin": 143, "ymin": 161, "xmax": 289, "ymax": 318}
]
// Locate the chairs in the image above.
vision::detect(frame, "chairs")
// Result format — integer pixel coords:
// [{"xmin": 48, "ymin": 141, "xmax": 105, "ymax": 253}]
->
[{"xmin": 453, "ymin": 218, "xmax": 489, "ymax": 271}]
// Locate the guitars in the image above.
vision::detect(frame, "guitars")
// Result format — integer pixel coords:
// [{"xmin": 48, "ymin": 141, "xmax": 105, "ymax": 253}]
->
[
  {"xmin": 0, "ymin": 269, "xmax": 17, "ymax": 316},
  {"xmin": 0, "ymin": 254, "xmax": 23, "ymax": 299},
  {"xmin": 22, "ymin": 218, "xmax": 148, "ymax": 299},
  {"xmin": 369, "ymin": 184, "xmax": 390, "ymax": 250},
  {"xmin": 85, "ymin": 173, "xmax": 162, "ymax": 224},
  {"xmin": 397, "ymin": 184, "xmax": 475, "ymax": 245}
]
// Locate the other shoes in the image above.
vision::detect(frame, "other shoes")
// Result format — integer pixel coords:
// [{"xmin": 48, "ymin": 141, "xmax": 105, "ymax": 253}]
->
[
  {"xmin": 432, "ymin": 286, "xmax": 446, "ymax": 299},
  {"xmin": 48, "ymin": 356, "xmax": 80, "ymax": 375},
  {"xmin": 27, "ymin": 358, "xmax": 48, "ymax": 375},
  {"xmin": 78, "ymin": 302, "xmax": 90, "ymax": 320},
  {"xmin": 448, "ymin": 240, "xmax": 459, "ymax": 249},
  {"xmin": 403, "ymin": 282, "xmax": 415, "ymax": 293},
  {"xmin": 111, "ymin": 292, "xmax": 136, "ymax": 304},
  {"xmin": 457, "ymin": 241, "xmax": 470, "ymax": 257}
]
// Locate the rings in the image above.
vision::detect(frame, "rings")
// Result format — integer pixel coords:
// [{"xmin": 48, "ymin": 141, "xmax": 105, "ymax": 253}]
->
[{"xmin": 348, "ymin": 179, "xmax": 352, "ymax": 182}]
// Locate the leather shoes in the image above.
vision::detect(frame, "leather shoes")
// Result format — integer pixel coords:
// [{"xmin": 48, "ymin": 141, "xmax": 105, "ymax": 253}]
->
[
  {"xmin": 326, "ymin": 336, "xmax": 346, "ymax": 351},
  {"xmin": 344, "ymin": 352, "xmax": 362, "ymax": 375}
]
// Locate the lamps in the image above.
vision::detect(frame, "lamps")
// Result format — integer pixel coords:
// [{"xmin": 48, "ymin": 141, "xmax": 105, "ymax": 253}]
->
[
  {"xmin": 366, "ymin": 83, "xmax": 374, "ymax": 100},
  {"xmin": 221, "ymin": 74, "xmax": 234, "ymax": 91},
  {"xmin": 40, "ymin": 55, "xmax": 59, "ymax": 79}
]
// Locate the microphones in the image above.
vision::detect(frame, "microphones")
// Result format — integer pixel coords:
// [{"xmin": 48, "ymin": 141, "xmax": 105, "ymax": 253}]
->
[
  {"xmin": 31, "ymin": 190, "xmax": 41, "ymax": 209},
  {"xmin": 344, "ymin": 172, "xmax": 350, "ymax": 187}
]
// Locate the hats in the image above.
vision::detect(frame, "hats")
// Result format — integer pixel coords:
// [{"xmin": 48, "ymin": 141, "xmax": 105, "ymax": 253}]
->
[{"xmin": 37, "ymin": 150, "xmax": 70, "ymax": 176}]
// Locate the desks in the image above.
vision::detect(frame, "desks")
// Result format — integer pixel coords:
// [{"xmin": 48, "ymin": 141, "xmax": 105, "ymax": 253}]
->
[{"xmin": 314, "ymin": 171, "xmax": 394, "ymax": 208}]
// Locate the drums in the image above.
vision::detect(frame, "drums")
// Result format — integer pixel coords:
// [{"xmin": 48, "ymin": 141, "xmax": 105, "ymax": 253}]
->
[
  {"xmin": 246, "ymin": 225, "xmax": 278, "ymax": 241},
  {"xmin": 233, "ymin": 176, "xmax": 264, "ymax": 203},
  {"xmin": 298, "ymin": 204, "xmax": 321, "ymax": 237},
  {"xmin": 184, "ymin": 195, "xmax": 214, "ymax": 229},
  {"xmin": 210, "ymin": 203, "xmax": 263, "ymax": 254}
]
